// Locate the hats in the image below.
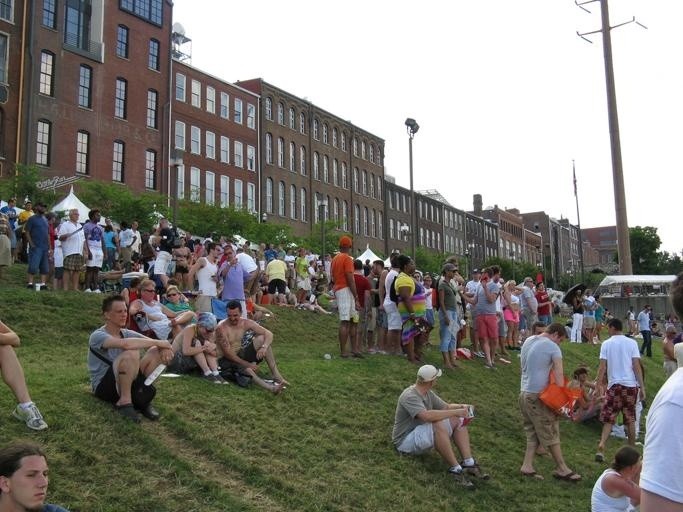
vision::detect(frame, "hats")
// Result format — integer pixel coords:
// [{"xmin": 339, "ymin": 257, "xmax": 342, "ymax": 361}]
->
[
  {"xmin": 440, "ymin": 263, "xmax": 459, "ymax": 272},
  {"xmin": 339, "ymin": 236, "xmax": 353, "ymax": 249},
  {"xmin": 417, "ymin": 364, "xmax": 444, "ymax": 382}
]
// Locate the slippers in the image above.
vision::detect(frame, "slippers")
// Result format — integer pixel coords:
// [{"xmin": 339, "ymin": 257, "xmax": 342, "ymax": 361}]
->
[
  {"xmin": 552, "ymin": 470, "xmax": 582, "ymax": 483},
  {"xmin": 518, "ymin": 468, "xmax": 544, "ymax": 481}
]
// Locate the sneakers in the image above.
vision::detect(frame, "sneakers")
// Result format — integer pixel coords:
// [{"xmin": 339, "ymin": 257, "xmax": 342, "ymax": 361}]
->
[
  {"xmin": 594, "ymin": 445, "xmax": 606, "ymax": 463},
  {"xmin": 212, "ymin": 369, "xmax": 229, "ymax": 385},
  {"xmin": 449, "ymin": 468, "xmax": 475, "ymax": 491},
  {"xmin": 340, "ymin": 344, "xmax": 521, "ymax": 371},
  {"xmin": 138, "ymin": 402, "xmax": 159, "ymax": 421},
  {"xmin": 204, "ymin": 371, "xmax": 221, "ymax": 385},
  {"xmin": 462, "ymin": 460, "xmax": 491, "ymax": 481},
  {"xmin": 27, "ymin": 283, "xmax": 101, "ymax": 294},
  {"xmin": 113, "ymin": 403, "xmax": 141, "ymax": 423},
  {"xmin": 12, "ymin": 401, "xmax": 49, "ymax": 431}
]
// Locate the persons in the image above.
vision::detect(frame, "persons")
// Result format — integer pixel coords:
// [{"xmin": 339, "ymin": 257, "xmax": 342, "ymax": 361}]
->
[
  {"xmin": 1, "ymin": 195, "xmax": 331, "ymax": 315},
  {"xmin": 387, "ymin": 362, "xmax": 489, "ymax": 493},
  {"xmin": 0, "ymin": 438, "xmax": 66, "ymax": 512},
  {"xmin": 121, "ymin": 276, "xmax": 293, "ymax": 396},
  {"xmin": 517, "ymin": 323, "xmax": 583, "ymax": 484},
  {"xmin": 594, "ymin": 316, "xmax": 647, "ymax": 462},
  {"xmin": 621, "ymin": 284, "xmax": 683, "ymax": 374},
  {"xmin": 568, "ymin": 360, "xmax": 647, "ymax": 421},
  {"xmin": 85, "ymin": 294, "xmax": 178, "ymax": 421},
  {"xmin": 589, "ymin": 445, "xmax": 643, "ymax": 512},
  {"xmin": 332, "ymin": 235, "xmax": 612, "ymax": 371},
  {"xmin": 0, "ymin": 320, "xmax": 47, "ymax": 430},
  {"xmin": 532, "ymin": 323, "xmax": 552, "ymax": 458},
  {"xmin": 639, "ymin": 269, "xmax": 683, "ymax": 512}
]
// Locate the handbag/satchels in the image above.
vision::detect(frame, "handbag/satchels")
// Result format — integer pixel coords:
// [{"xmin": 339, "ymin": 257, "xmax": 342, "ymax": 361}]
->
[{"xmin": 535, "ymin": 367, "xmax": 586, "ymax": 424}]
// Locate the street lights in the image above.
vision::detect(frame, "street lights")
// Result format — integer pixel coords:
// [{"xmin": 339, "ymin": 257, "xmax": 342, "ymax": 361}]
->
[
  {"xmin": 261, "ymin": 211, "xmax": 265, "ymax": 223},
  {"xmin": 552, "ymin": 220, "xmax": 559, "ymax": 290},
  {"xmin": 464, "ymin": 239, "xmax": 473, "ymax": 280},
  {"xmin": 316, "ymin": 194, "xmax": 328, "ymax": 263},
  {"xmin": 464, "ymin": 249, "xmax": 469, "ymax": 282},
  {"xmin": 167, "ymin": 146, "xmax": 183, "ymax": 227},
  {"xmin": 401, "ymin": 117, "xmax": 420, "ymax": 262},
  {"xmin": 509, "ymin": 249, "xmax": 514, "ymax": 281}
]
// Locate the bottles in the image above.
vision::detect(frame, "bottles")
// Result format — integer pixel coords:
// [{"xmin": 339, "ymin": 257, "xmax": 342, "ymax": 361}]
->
[{"xmin": 353, "ymin": 311, "xmax": 359, "ymax": 323}]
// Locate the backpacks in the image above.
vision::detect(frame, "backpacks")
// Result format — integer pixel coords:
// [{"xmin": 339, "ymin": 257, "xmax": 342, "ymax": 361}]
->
[{"xmin": 389, "ymin": 277, "xmax": 400, "ymax": 304}]
